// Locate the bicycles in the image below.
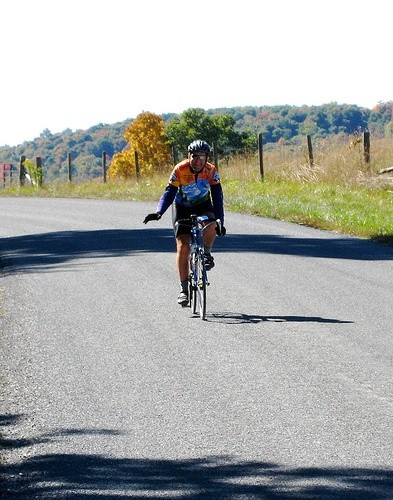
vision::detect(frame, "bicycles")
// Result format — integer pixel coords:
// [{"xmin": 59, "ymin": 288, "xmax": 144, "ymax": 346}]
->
[{"xmin": 173, "ymin": 214, "xmax": 222, "ymax": 321}]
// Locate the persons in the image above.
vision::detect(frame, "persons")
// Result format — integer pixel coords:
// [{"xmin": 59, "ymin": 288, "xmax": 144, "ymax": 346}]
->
[{"xmin": 142, "ymin": 141, "xmax": 226, "ymax": 305}]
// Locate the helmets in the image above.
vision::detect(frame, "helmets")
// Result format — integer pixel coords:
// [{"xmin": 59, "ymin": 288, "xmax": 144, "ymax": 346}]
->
[{"xmin": 187, "ymin": 140, "xmax": 210, "ymax": 155}]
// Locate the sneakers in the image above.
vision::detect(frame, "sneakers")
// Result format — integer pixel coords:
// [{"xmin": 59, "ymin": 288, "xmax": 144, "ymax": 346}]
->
[
  {"xmin": 178, "ymin": 292, "xmax": 189, "ymax": 304},
  {"xmin": 202, "ymin": 254, "xmax": 214, "ymax": 271}
]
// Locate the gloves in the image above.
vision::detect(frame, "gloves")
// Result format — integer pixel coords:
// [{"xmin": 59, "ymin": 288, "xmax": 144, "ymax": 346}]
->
[
  {"xmin": 143, "ymin": 214, "xmax": 161, "ymax": 224},
  {"xmin": 215, "ymin": 224, "xmax": 226, "ymax": 237}
]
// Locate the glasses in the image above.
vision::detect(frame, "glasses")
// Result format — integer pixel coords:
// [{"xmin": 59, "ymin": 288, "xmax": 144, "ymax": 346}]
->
[{"xmin": 190, "ymin": 153, "xmax": 206, "ymax": 160}]
